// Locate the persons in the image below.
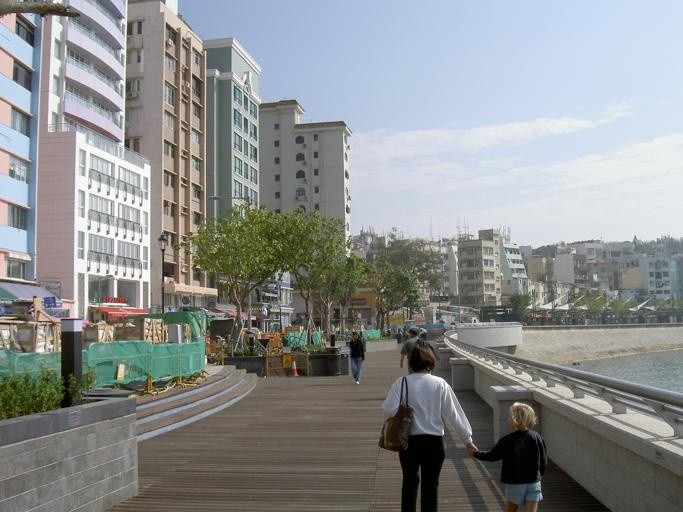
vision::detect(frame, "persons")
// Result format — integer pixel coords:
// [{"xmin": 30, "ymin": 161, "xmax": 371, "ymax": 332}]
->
[
  {"xmin": 400, "ymin": 328, "xmax": 420, "ymax": 375},
  {"xmin": 382, "ymin": 339, "xmax": 478, "ymax": 512},
  {"xmin": 469, "ymin": 400, "xmax": 548, "ymax": 512},
  {"xmin": 348, "ymin": 331, "xmax": 365, "ymax": 385}
]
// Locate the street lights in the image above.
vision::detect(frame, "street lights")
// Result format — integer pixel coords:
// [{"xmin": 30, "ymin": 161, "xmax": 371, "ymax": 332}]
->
[
  {"xmin": 157, "ymin": 234, "xmax": 168, "ymax": 314},
  {"xmin": 277, "ymin": 277, "xmax": 282, "ymax": 331}
]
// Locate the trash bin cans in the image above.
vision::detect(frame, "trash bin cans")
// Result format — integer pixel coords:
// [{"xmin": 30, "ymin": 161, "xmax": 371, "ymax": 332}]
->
[
  {"xmin": 396, "ymin": 333, "xmax": 401, "ymax": 344},
  {"xmin": 362, "ymin": 341, "xmax": 366, "ymax": 352},
  {"xmin": 341, "ymin": 354, "xmax": 348, "ymax": 374},
  {"xmin": 258, "ymin": 339, "xmax": 269, "ymax": 351}
]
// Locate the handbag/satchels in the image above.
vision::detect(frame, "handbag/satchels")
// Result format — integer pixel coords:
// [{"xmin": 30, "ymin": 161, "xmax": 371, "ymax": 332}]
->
[{"xmin": 378, "ymin": 405, "xmax": 414, "ymax": 452}]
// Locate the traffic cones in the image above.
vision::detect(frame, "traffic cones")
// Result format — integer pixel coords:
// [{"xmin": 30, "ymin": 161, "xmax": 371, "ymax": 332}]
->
[{"xmin": 286, "ymin": 355, "xmax": 299, "ymax": 377}]
